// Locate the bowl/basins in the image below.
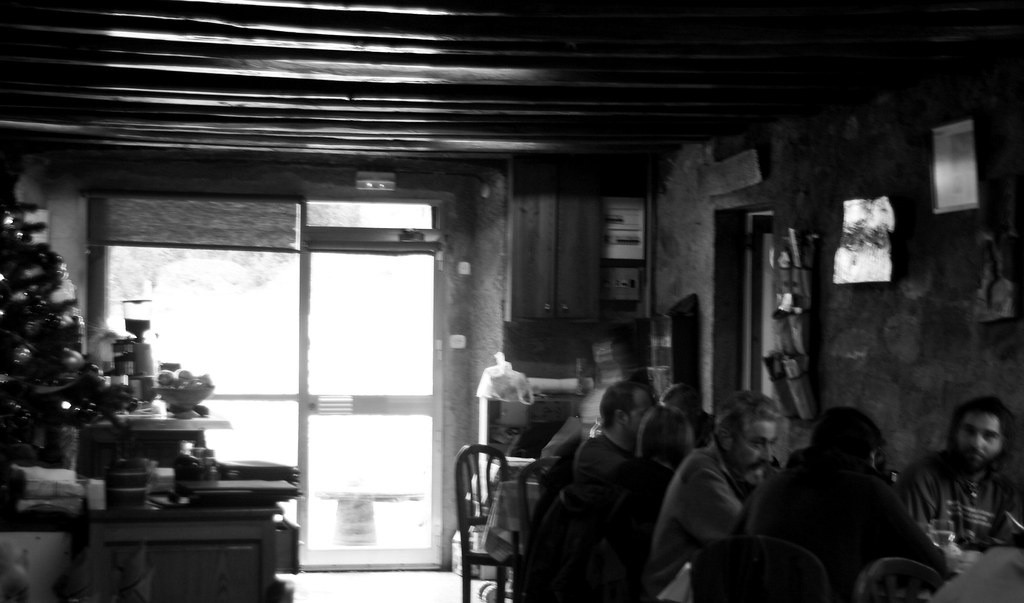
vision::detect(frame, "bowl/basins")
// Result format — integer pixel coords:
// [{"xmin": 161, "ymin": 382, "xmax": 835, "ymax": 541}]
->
[{"xmin": 152, "ymin": 386, "xmax": 215, "ymax": 417}]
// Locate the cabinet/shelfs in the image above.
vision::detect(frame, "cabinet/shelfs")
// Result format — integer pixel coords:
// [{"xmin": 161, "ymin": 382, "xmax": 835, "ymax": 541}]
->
[{"xmin": 500, "ymin": 155, "xmax": 655, "ymax": 327}]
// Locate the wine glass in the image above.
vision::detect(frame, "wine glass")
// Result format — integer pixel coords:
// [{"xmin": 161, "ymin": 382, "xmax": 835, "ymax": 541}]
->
[{"xmin": 931, "ymin": 520, "xmax": 955, "ymax": 547}]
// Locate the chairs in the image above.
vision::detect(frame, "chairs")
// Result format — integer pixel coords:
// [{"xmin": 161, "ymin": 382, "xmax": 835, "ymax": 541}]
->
[
  {"xmin": 692, "ymin": 533, "xmax": 948, "ymax": 603},
  {"xmin": 452, "ymin": 444, "xmax": 575, "ymax": 603}
]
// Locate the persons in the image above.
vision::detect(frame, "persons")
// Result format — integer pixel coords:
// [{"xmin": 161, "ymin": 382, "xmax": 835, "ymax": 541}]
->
[
  {"xmin": 520, "ymin": 383, "xmax": 654, "ymax": 603},
  {"xmin": 514, "ymin": 399, "xmax": 555, "ymax": 458},
  {"xmin": 642, "ymin": 389, "xmax": 786, "ymax": 603},
  {"xmin": 526, "ymin": 390, "xmax": 604, "ymax": 561},
  {"xmin": 604, "ymin": 402, "xmax": 694, "ymax": 558},
  {"xmin": 659, "ymin": 383, "xmax": 714, "ymax": 447},
  {"xmin": 735, "ymin": 406, "xmax": 957, "ymax": 603},
  {"xmin": 895, "ymin": 395, "xmax": 1024, "ymax": 551}
]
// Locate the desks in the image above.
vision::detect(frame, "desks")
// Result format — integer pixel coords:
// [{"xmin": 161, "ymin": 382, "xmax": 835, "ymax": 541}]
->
[
  {"xmin": 76, "ymin": 404, "xmax": 206, "ymax": 469},
  {"xmin": 74, "ymin": 489, "xmax": 298, "ymax": 603}
]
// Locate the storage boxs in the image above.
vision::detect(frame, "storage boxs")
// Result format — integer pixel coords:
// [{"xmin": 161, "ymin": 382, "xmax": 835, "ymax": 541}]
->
[{"xmin": 598, "ymin": 195, "xmax": 647, "ymax": 263}]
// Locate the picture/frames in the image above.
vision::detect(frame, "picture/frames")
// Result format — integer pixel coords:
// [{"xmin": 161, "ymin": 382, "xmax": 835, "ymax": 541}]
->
[{"xmin": 923, "ymin": 116, "xmax": 987, "ymax": 222}]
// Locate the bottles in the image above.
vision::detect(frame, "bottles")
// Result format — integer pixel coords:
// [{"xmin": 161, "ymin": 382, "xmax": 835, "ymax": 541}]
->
[{"xmin": 191, "ymin": 448, "xmax": 219, "ymax": 480}]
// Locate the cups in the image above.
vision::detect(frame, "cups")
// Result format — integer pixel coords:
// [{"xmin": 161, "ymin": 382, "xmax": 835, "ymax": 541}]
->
[{"xmin": 89, "ymin": 480, "xmax": 105, "ymax": 510}]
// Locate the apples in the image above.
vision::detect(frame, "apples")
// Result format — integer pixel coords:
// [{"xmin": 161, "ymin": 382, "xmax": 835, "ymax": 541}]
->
[{"xmin": 156, "ymin": 368, "xmax": 212, "ymax": 389}]
